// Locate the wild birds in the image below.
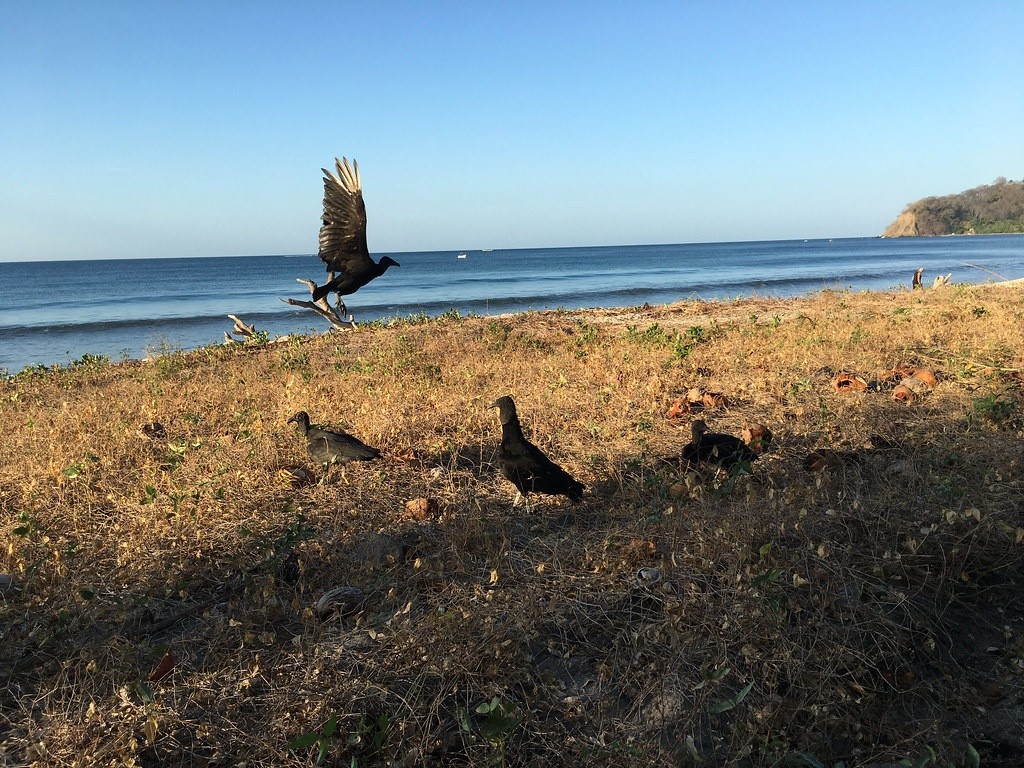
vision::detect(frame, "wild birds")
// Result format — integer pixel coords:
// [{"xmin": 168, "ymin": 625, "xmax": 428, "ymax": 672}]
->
[
  {"xmin": 287, "ymin": 411, "xmax": 385, "ymax": 485},
  {"xmin": 311, "ymin": 156, "xmax": 401, "ymax": 303},
  {"xmin": 680, "ymin": 418, "xmax": 767, "ymax": 482},
  {"xmin": 486, "ymin": 396, "xmax": 587, "ymax": 515}
]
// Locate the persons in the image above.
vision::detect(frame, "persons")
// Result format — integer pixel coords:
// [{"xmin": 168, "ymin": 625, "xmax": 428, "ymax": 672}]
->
[{"xmin": 912, "ymin": 267, "xmax": 924, "ymax": 289}]
